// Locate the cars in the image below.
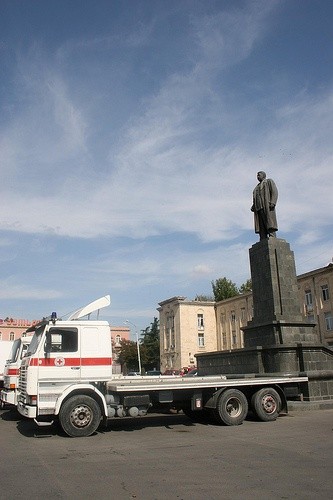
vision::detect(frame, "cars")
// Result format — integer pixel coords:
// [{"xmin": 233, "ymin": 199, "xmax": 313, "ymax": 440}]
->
[{"xmin": 129, "ymin": 366, "xmax": 199, "ymax": 376}]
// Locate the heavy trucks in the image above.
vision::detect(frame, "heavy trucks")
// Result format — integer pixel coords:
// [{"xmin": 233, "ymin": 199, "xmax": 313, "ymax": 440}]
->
[
  {"xmin": 16, "ymin": 313, "xmax": 308, "ymax": 437},
  {"xmin": 3, "ymin": 336, "xmax": 34, "ymax": 408}
]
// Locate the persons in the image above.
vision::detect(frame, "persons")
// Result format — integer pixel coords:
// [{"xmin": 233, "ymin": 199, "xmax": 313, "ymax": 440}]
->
[{"xmin": 251, "ymin": 171, "xmax": 279, "ymax": 238}]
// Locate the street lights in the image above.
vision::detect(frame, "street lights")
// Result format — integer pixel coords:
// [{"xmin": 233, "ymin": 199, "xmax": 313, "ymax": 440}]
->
[{"xmin": 124, "ymin": 318, "xmax": 142, "ymax": 376}]
